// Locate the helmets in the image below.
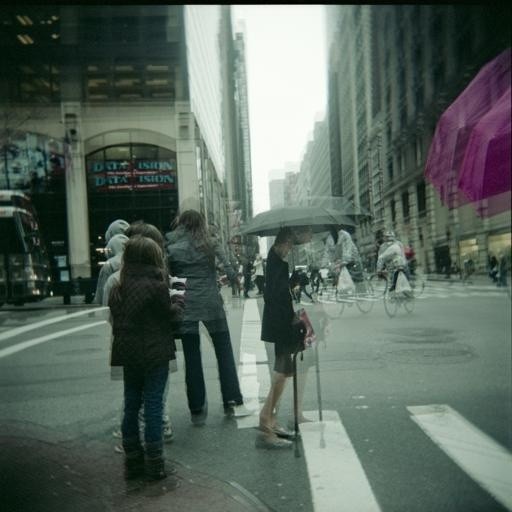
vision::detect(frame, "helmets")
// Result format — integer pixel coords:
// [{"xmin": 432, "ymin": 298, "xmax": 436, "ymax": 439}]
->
[{"xmin": 383, "ymin": 231, "xmax": 395, "ymax": 237}]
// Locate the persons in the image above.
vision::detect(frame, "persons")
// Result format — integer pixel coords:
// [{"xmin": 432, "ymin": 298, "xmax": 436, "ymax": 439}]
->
[{"xmin": 94, "ymin": 209, "xmax": 414, "ymax": 481}]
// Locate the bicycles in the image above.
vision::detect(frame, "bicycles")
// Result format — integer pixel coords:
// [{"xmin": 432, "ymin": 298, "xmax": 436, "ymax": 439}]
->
[{"xmin": 313, "ymin": 269, "xmax": 428, "ymax": 320}]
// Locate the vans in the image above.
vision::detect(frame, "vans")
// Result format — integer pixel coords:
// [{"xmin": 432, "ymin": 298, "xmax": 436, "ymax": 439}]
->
[{"xmin": 0, "ymin": 204, "xmax": 55, "ymax": 307}]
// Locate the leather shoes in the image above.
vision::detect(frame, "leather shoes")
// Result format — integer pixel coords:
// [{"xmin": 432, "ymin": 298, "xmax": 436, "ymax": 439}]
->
[
  {"xmin": 260, "ymin": 421, "xmax": 295, "ymax": 436},
  {"xmin": 255, "ymin": 435, "xmax": 293, "ymax": 449}
]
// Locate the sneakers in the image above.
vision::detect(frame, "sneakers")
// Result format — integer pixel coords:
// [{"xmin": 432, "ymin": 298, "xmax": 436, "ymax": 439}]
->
[
  {"xmin": 144, "ymin": 478, "xmax": 180, "ymax": 496},
  {"xmin": 161, "ymin": 413, "xmax": 175, "ymax": 443}
]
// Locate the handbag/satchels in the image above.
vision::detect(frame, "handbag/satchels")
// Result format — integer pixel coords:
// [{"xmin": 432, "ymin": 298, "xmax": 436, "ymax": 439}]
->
[
  {"xmin": 288, "ymin": 319, "xmax": 306, "ymax": 352},
  {"xmin": 297, "ymin": 308, "xmax": 317, "ymax": 347}
]
